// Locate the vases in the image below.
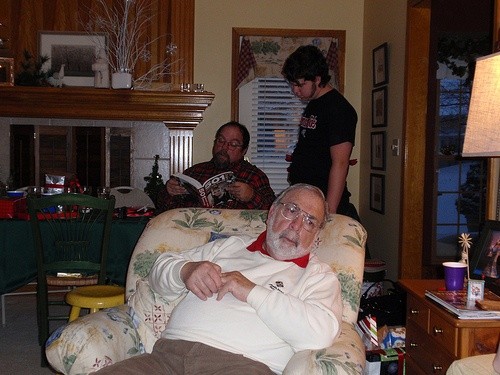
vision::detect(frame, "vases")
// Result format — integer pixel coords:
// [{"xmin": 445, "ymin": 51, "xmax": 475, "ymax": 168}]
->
[{"xmin": 112, "ymin": 73, "xmax": 132, "ymax": 89}]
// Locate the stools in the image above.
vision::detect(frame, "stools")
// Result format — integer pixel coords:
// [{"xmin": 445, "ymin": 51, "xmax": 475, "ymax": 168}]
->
[{"xmin": 66, "ymin": 285, "xmax": 126, "ymax": 325}]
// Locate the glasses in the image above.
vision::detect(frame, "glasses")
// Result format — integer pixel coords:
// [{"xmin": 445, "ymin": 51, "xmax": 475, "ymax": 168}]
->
[
  {"xmin": 277, "ymin": 201, "xmax": 323, "ymax": 233},
  {"xmin": 285, "ymin": 79, "xmax": 315, "ymax": 88},
  {"xmin": 215, "ymin": 137, "xmax": 245, "ymax": 151}
]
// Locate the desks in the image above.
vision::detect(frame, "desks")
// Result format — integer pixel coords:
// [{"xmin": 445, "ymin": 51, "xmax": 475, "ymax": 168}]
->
[{"xmin": 0, "ymin": 215, "xmax": 156, "ymax": 327}]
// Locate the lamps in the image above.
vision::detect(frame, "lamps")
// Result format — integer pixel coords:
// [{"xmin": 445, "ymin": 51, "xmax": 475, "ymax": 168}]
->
[{"xmin": 461, "ymin": 51, "xmax": 500, "ymax": 221}]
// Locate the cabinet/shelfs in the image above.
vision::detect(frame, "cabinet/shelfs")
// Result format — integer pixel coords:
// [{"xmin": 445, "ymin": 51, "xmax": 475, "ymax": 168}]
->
[{"xmin": 399, "ymin": 279, "xmax": 500, "ymax": 375}]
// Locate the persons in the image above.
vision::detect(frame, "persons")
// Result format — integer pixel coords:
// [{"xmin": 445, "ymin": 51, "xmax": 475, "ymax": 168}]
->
[
  {"xmin": 156, "ymin": 121, "xmax": 277, "ymax": 209},
  {"xmin": 446, "ymin": 340, "xmax": 500, "ymax": 375},
  {"xmin": 90, "ymin": 183, "xmax": 343, "ymax": 375},
  {"xmin": 282, "ymin": 45, "xmax": 357, "ymax": 214}
]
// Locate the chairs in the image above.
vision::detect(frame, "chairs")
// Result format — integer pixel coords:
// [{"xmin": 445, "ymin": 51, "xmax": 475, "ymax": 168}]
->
[{"xmin": 28, "ymin": 193, "xmax": 116, "ymax": 367}]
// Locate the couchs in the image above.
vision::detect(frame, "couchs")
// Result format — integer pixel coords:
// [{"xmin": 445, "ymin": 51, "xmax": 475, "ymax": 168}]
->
[{"xmin": 44, "ymin": 209, "xmax": 366, "ymax": 375}]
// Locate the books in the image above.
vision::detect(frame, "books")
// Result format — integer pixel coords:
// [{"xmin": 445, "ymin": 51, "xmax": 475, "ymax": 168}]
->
[
  {"xmin": 171, "ymin": 171, "xmax": 236, "ymax": 208},
  {"xmin": 425, "ymin": 289, "xmax": 500, "ymax": 320}
]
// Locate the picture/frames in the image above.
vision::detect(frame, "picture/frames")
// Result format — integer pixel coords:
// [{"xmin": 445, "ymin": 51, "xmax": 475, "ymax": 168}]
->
[
  {"xmin": 370, "ymin": 131, "xmax": 386, "ymax": 171},
  {"xmin": 371, "ymin": 86, "xmax": 388, "ymax": 128},
  {"xmin": 369, "ymin": 172, "xmax": 386, "ymax": 216},
  {"xmin": 466, "ymin": 219, "xmax": 500, "ymax": 297},
  {"xmin": 373, "ymin": 42, "xmax": 389, "ymax": 88},
  {"xmin": 38, "ymin": 31, "xmax": 109, "ymax": 87}
]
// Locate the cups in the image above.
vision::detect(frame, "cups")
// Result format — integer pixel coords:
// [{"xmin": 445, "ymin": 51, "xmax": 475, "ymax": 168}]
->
[
  {"xmin": 442, "ymin": 262, "xmax": 468, "ymax": 291},
  {"xmin": 7, "ymin": 191, "xmax": 24, "ymax": 198},
  {"xmin": 82, "ymin": 185, "xmax": 110, "ymax": 199},
  {"xmin": 95, "ymin": 70, "xmax": 110, "ymax": 88}
]
